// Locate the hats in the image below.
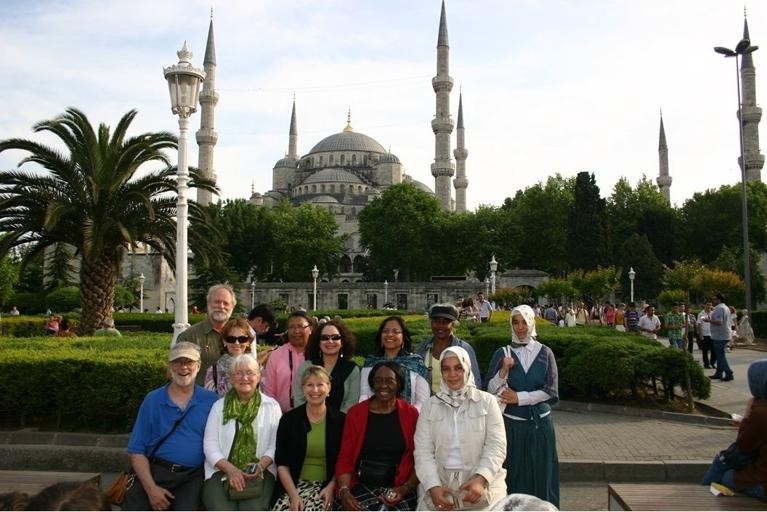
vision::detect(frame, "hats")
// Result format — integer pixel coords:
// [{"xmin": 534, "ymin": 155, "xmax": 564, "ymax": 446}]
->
[
  {"xmin": 167, "ymin": 340, "xmax": 202, "ymax": 362},
  {"xmin": 438, "ymin": 344, "xmax": 476, "ymax": 393},
  {"xmin": 509, "ymin": 304, "xmax": 538, "ymax": 344},
  {"xmin": 428, "ymin": 302, "xmax": 460, "ymax": 321}
]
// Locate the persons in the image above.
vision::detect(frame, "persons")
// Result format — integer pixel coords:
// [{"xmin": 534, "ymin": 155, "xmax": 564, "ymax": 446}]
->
[
  {"xmin": 9, "ymin": 306, "xmax": 19, "ymax": 316},
  {"xmin": 120, "ymin": 282, "xmax": 560, "ymax": 510},
  {"xmin": 533, "ymin": 298, "xmax": 660, "ymax": 340},
  {"xmin": 52, "ymin": 319, "xmax": 76, "ymax": 337},
  {"xmin": 22, "ymin": 481, "xmax": 112, "ymax": 511},
  {"xmin": 701, "ymin": 361, "xmax": 767, "ymax": 501},
  {"xmin": 46, "ymin": 314, "xmax": 60, "ymax": 336},
  {"xmin": 93, "ymin": 317, "xmax": 122, "ymax": 337},
  {"xmin": 664, "ymin": 294, "xmax": 754, "ymax": 382}
]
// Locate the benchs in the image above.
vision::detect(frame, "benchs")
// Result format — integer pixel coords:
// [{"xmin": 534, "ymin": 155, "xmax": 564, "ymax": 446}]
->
[{"xmin": 607, "ymin": 483, "xmax": 767, "ymax": 511}]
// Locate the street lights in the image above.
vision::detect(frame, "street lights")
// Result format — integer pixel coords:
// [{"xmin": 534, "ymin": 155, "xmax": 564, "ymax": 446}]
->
[
  {"xmin": 382, "ymin": 278, "xmax": 389, "ymax": 308},
  {"xmin": 711, "ymin": 38, "xmax": 761, "ymax": 339},
  {"xmin": 157, "ymin": 41, "xmax": 206, "ymax": 353},
  {"xmin": 627, "ymin": 267, "xmax": 637, "ymax": 304},
  {"xmin": 488, "ymin": 256, "xmax": 498, "ymax": 313},
  {"xmin": 137, "ymin": 271, "xmax": 146, "ymax": 313},
  {"xmin": 250, "ymin": 281, "xmax": 256, "ymax": 311},
  {"xmin": 484, "ymin": 277, "xmax": 490, "ymax": 305},
  {"xmin": 311, "ymin": 264, "xmax": 320, "ymax": 311}
]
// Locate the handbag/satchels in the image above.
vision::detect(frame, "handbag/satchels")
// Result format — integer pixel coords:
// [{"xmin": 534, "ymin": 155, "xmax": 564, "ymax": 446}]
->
[
  {"xmin": 101, "ymin": 470, "xmax": 133, "ymax": 507},
  {"xmin": 492, "ymin": 344, "xmax": 516, "ymax": 415},
  {"xmin": 222, "ymin": 463, "xmax": 265, "ymax": 501}
]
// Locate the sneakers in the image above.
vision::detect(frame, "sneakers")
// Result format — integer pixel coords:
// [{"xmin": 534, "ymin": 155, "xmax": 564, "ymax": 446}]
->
[
  {"xmin": 708, "ymin": 373, "xmax": 735, "ymax": 382},
  {"xmin": 704, "ymin": 364, "xmax": 717, "ymax": 370}
]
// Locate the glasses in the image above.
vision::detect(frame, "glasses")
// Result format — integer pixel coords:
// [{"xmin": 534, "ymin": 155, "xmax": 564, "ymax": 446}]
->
[
  {"xmin": 286, "ymin": 323, "xmax": 310, "ymax": 330},
  {"xmin": 222, "ymin": 334, "xmax": 251, "ymax": 345},
  {"xmin": 319, "ymin": 333, "xmax": 343, "ymax": 342}
]
